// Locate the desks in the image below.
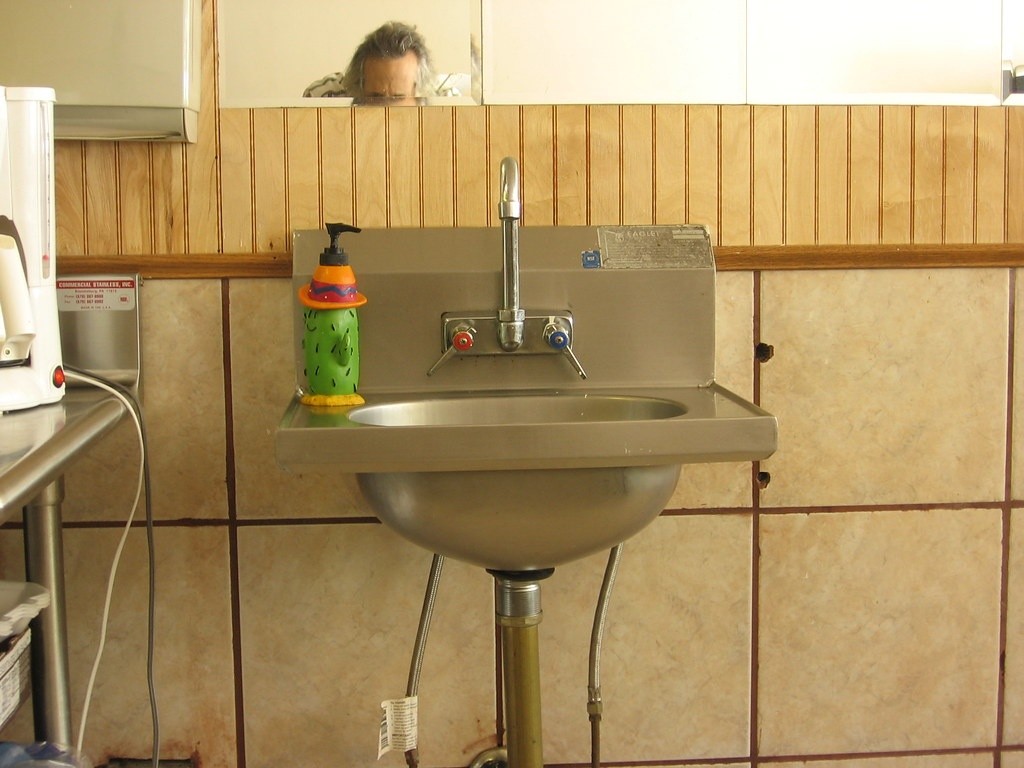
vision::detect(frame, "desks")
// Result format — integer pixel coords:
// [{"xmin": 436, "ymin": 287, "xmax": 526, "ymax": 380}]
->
[{"xmin": 0, "ymin": 388, "xmax": 144, "ymax": 745}]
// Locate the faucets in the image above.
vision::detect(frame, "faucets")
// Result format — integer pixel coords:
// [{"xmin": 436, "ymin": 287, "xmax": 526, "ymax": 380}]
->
[{"xmin": 498, "ymin": 157, "xmax": 526, "ymax": 348}]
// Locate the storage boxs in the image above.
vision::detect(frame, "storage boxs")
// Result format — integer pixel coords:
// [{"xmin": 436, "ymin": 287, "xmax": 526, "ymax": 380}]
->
[{"xmin": 0, "ymin": 628, "xmax": 33, "ymax": 733}]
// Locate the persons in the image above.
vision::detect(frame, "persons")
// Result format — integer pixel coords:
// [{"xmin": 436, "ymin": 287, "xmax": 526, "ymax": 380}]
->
[{"xmin": 339, "ymin": 22, "xmax": 434, "ymax": 96}]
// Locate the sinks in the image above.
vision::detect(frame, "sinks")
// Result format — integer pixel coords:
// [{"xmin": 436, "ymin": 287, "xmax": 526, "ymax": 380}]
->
[{"xmin": 270, "ymin": 385, "xmax": 779, "ymax": 568}]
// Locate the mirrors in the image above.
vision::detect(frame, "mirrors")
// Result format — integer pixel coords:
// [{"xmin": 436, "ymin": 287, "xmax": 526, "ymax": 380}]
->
[{"xmin": 216, "ymin": 0, "xmax": 482, "ymax": 109}]
